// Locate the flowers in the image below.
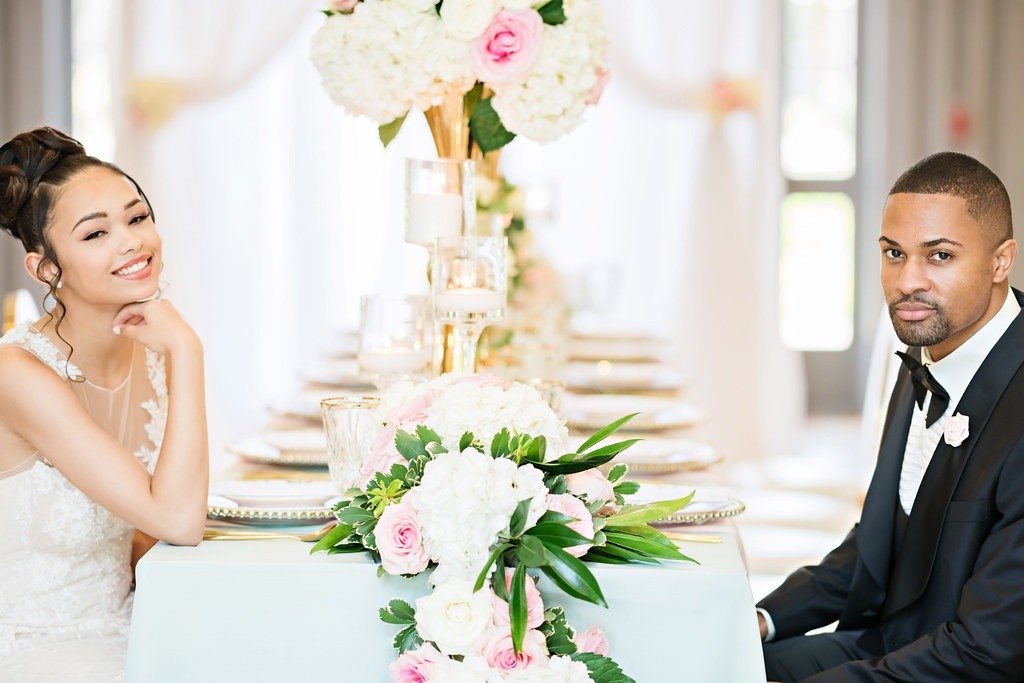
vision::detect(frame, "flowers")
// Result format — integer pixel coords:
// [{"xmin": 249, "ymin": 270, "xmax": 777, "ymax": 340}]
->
[
  {"xmin": 308, "ymin": 0, "xmax": 613, "ymax": 161},
  {"xmin": 307, "ymin": 369, "xmax": 702, "ymax": 683},
  {"xmin": 942, "ymin": 413, "xmax": 971, "ymax": 447}
]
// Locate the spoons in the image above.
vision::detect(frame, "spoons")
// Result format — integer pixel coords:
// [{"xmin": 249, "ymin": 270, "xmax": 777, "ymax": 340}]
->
[{"xmin": 203, "ymin": 524, "xmax": 336, "ymax": 541}]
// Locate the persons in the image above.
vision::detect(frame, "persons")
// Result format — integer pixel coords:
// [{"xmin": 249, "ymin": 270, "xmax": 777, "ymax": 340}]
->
[
  {"xmin": 0, "ymin": 125, "xmax": 208, "ymax": 683},
  {"xmin": 756, "ymin": 152, "xmax": 1024, "ymax": 683}
]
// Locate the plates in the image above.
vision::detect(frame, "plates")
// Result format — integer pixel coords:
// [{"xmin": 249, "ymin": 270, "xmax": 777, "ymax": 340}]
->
[
  {"xmin": 567, "ymin": 396, "xmax": 696, "ymax": 429},
  {"xmin": 209, "ymin": 479, "xmax": 339, "ymax": 520},
  {"xmin": 237, "ymin": 429, "xmax": 325, "ymax": 467},
  {"xmin": 621, "ymin": 482, "xmax": 747, "ymax": 524},
  {"xmin": 596, "ymin": 436, "xmax": 724, "ymax": 473},
  {"xmin": 568, "ymin": 361, "xmax": 685, "ymax": 392}
]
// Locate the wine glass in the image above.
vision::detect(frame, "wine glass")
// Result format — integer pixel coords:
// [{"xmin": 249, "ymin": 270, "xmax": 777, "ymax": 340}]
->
[
  {"xmin": 357, "ymin": 295, "xmax": 435, "ymax": 416},
  {"xmin": 435, "ymin": 237, "xmax": 510, "ymax": 374}
]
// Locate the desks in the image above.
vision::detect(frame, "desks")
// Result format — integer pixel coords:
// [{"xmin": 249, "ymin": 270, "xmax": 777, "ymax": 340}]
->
[{"xmin": 126, "ymin": 341, "xmax": 767, "ymax": 683}]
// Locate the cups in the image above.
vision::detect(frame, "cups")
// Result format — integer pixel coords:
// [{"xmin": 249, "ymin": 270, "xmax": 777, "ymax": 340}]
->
[
  {"xmin": 403, "ymin": 156, "xmax": 473, "ymax": 256},
  {"xmin": 322, "ymin": 397, "xmax": 379, "ymax": 494}
]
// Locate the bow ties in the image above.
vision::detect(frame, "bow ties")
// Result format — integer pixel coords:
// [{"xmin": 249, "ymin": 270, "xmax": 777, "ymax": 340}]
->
[{"xmin": 895, "ymin": 351, "xmax": 949, "ymax": 429}]
